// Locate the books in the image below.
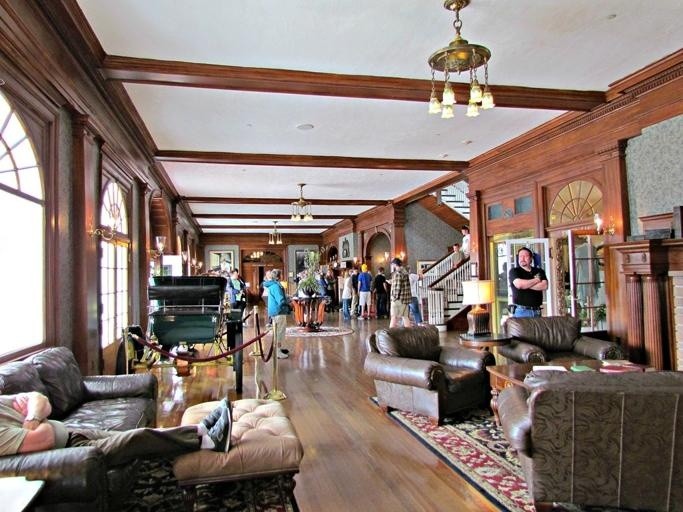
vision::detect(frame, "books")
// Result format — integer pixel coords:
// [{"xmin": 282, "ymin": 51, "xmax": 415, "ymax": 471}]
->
[{"xmin": 532, "ymin": 358, "xmax": 657, "ymax": 375}]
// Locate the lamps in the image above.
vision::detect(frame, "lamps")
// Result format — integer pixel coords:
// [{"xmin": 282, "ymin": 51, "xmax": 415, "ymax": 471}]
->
[
  {"xmin": 150, "ymin": 235, "xmax": 166, "ymax": 258},
  {"xmin": 87, "ymin": 212, "xmax": 119, "ymax": 241},
  {"xmin": 288, "ymin": 183, "xmax": 313, "ymax": 222},
  {"xmin": 459, "ymin": 278, "xmax": 498, "ymax": 335},
  {"xmin": 428, "ymin": 0, "xmax": 495, "ymax": 120},
  {"xmin": 384, "ymin": 250, "xmax": 405, "ymax": 264},
  {"xmin": 179, "ymin": 250, "xmax": 202, "ymax": 269},
  {"xmin": 589, "ymin": 210, "xmax": 616, "ymax": 236},
  {"xmin": 267, "ymin": 220, "xmax": 283, "ymax": 244}
]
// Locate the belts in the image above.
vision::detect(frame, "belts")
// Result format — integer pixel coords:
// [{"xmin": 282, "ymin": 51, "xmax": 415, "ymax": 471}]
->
[{"xmin": 518, "ymin": 305, "xmax": 544, "ymax": 311}]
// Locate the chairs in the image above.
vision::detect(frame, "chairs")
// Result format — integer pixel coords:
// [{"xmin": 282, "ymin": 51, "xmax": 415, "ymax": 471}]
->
[
  {"xmin": 489, "ymin": 370, "xmax": 682, "ymax": 511},
  {"xmin": 495, "ymin": 313, "xmax": 628, "ymax": 364},
  {"xmin": 362, "ymin": 321, "xmax": 494, "ymax": 428}
]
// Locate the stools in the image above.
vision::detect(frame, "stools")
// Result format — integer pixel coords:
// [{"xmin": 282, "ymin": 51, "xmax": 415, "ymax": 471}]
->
[{"xmin": 177, "ymin": 396, "xmax": 305, "ymax": 512}]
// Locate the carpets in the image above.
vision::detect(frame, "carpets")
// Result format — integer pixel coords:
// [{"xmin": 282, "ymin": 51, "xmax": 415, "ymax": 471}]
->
[
  {"xmin": 118, "ymin": 452, "xmax": 301, "ymax": 512},
  {"xmin": 369, "ymin": 395, "xmax": 532, "ymax": 511},
  {"xmin": 265, "ymin": 325, "xmax": 354, "ymax": 336}
]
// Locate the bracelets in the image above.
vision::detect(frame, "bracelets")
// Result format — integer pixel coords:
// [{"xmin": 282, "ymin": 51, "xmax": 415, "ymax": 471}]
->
[{"xmin": 23, "ymin": 415, "xmax": 42, "ymax": 422}]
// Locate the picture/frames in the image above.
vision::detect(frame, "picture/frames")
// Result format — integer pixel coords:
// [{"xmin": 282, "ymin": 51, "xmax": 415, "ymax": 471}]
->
[
  {"xmin": 415, "ymin": 259, "xmax": 437, "ymax": 275},
  {"xmin": 208, "ymin": 251, "xmax": 233, "ymax": 273},
  {"xmin": 294, "ymin": 250, "xmax": 316, "ymax": 285}
]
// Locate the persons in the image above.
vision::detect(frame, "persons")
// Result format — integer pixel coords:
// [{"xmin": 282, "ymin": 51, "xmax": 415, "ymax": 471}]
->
[
  {"xmin": 320, "ymin": 258, "xmax": 425, "ymax": 328},
  {"xmin": 261, "ymin": 268, "xmax": 293, "ymax": 360},
  {"xmin": 459, "ymin": 225, "xmax": 471, "ymax": 259},
  {"xmin": 0, "ymin": 389, "xmax": 235, "ymax": 469},
  {"xmin": 261, "ymin": 270, "xmax": 273, "ymax": 327},
  {"xmin": 450, "ymin": 244, "xmax": 465, "ymax": 279},
  {"xmin": 226, "ymin": 268, "xmax": 248, "ymax": 319},
  {"xmin": 507, "ymin": 246, "xmax": 548, "ymax": 317}
]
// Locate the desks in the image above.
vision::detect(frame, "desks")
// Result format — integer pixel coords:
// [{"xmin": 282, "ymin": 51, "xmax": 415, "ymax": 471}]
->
[
  {"xmin": 292, "ymin": 295, "xmax": 327, "ymax": 330},
  {"xmin": 458, "ymin": 336, "xmax": 516, "ymax": 354}
]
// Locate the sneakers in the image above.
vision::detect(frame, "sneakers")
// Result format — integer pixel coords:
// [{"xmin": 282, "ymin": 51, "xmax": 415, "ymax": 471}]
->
[
  {"xmin": 344, "ymin": 314, "xmax": 388, "ymax": 321},
  {"xmin": 276, "ymin": 346, "xmax": 289, "ymax": 359}
]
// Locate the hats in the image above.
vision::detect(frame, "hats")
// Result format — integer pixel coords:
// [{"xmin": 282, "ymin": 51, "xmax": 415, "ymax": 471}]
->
[
  {"xmin": 270, "ymin": 268, "xmax": 283, "ymax": 277},
  {"xmin": 388, "ymin": 258, "xmax": 403, "ymax": 267},
  {"xmin": 360, "ymin": 264, "xmax": 369, "ymax": 273}
]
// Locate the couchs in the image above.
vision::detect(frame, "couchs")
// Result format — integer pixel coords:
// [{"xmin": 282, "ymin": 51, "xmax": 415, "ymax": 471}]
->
[{"xmin": 0, "ymin": 343, "xmax": 161, "ymax": 509}]
